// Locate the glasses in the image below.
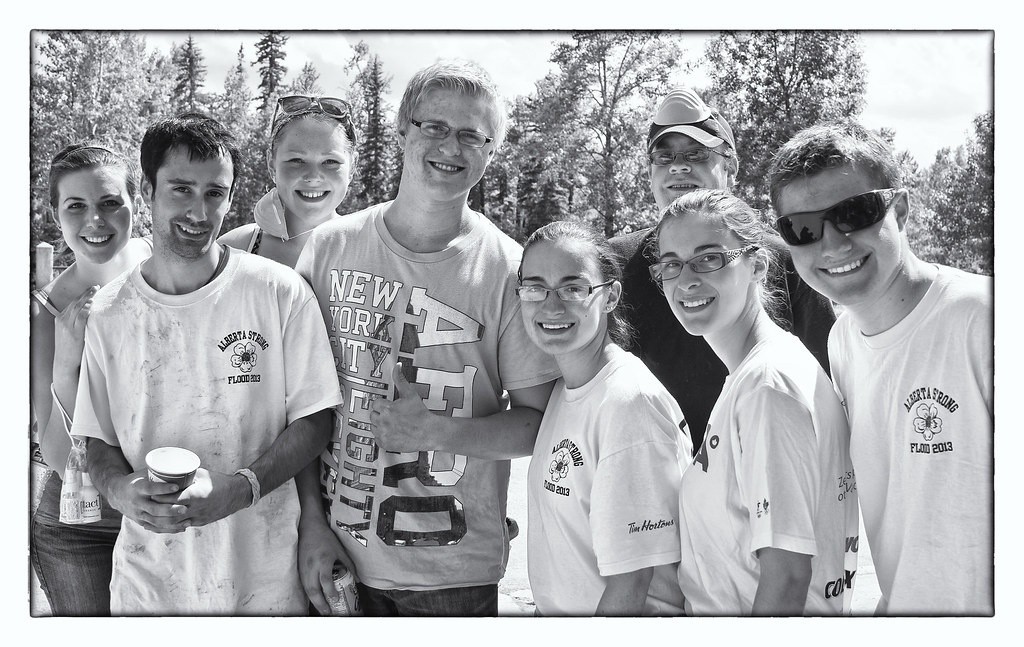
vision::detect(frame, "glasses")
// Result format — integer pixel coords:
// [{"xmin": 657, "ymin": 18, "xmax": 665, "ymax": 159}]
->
[
  {"xmin": 646, "ymin": 147, "xmax": 730, "ymax": 166},
  {"xmin": 775, "ymin": 187, "xmax": 896, "ymax": 246},
  {"xmin": 409, "ymin": 119, "xmax": 493, "ymax": 148},
  {"xmin": 514, "ymin": 280, "xmax": 615, "ymax": 302},
  {"xmin": 271, "ymin": 95, "xmax": 353, "ymax": 139},
  {"xmin": 647, "ymin": 245, "xmax": 759, "ymax": 281}
]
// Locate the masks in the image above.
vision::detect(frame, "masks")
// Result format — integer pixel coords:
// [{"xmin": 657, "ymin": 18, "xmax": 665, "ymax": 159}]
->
[{"xmin": 654, "ymin": 85, "xmax": 734, "ymax": 145}]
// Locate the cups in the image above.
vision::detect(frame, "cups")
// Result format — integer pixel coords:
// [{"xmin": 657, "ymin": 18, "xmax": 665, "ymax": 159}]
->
[{"xmin": 144, "ymin": 447, "xmax": 200, "ymax": 496}]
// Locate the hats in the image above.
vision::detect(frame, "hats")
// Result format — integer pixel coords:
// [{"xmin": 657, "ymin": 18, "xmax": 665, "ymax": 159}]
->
[{"xmin": 646, "ymin": 104, "xmax": 736, "ymax": 160}]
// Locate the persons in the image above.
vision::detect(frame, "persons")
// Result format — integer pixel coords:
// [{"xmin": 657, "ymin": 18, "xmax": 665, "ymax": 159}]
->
[
  {"xmin": 214, "ymin": 94, "xmax": 359, "ymax": 274},
  {"xmin": 773, "ymin": 120, "xmax": 993, "ymax": 615},
  {"xmin": 518, "ymin": 211, "xmax": 694, "ymax": 616},
  {"xmin": 658, "ymin": 189, "xmax": 859, "ymax": 617},
  {"xmin": 292, "ymin": 54, "xmax": 564, "ymax": 617},
  {"xmin": 607, "ymin": 89, "xmax": 837, "ymax": 460},
  {"xmin": 31, "ymin": 112, "xmax": 344, "ymax": 617}
]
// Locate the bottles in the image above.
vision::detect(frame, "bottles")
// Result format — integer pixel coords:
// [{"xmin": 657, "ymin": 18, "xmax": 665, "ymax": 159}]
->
[
  {"xmin": 80, "ymin": 472, "xmax": 102, "ymax": 523},
  {"xmin": 60, "ymin": 469, "xmax": 83, "ymax": 524}
]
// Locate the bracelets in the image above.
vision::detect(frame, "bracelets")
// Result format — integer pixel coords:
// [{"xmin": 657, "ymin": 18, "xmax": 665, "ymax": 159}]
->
[{"xmin": 235, "ymin": 469, "xmax": 261, "ymax": 509}]
